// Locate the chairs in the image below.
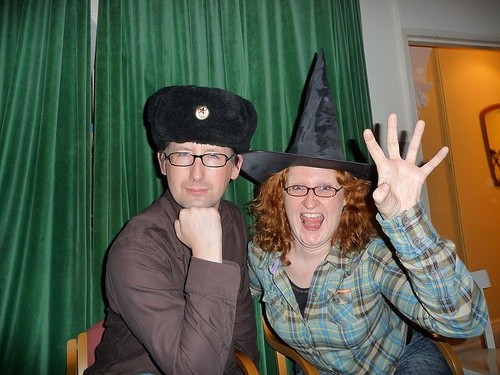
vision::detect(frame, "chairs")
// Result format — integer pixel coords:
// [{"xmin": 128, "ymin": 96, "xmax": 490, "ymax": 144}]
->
[
  {"xmin": 67, "ymin": 319, "xmax": 258, "ymax": 375},
  {"xmin": 263, "ymin": 314, "xmax": 464, "ymax": 375},
  {"xmin": 453, "ymin": 269, "xmax": 500, "ymax": 374}
]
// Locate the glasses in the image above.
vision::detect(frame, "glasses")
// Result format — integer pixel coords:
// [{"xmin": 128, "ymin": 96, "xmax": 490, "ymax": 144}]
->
[
  {"xmin": 283, "ymin": 182, "xmax": 343, "ymax": 198},
  {"xmin": 166, "ymin": 152, "xmax": 235, "ymax": 168}
]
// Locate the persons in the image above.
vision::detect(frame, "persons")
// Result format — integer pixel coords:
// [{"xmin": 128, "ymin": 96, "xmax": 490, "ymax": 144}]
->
[
  {"xmin": 243, "ymin": 47, "xmax": 488, "ymax": 375},
  {"xmin": 82, "ymin": 85, "xmax": 258, "ymax": 375}
]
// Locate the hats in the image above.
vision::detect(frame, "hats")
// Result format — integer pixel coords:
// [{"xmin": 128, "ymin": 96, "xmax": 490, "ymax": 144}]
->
[
  {"xmin": 240, "ymin": 48, "xmax": 379, "ymax": 184},
  {"xmin": 142, "ymin": 85, "xmax": 257, "ymax": 153}
]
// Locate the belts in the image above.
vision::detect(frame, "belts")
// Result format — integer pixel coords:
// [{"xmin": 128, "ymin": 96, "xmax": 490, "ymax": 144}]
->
[{"xmin": 406, "ymin": 325, "xmax": 413, "ymax": 345}]
